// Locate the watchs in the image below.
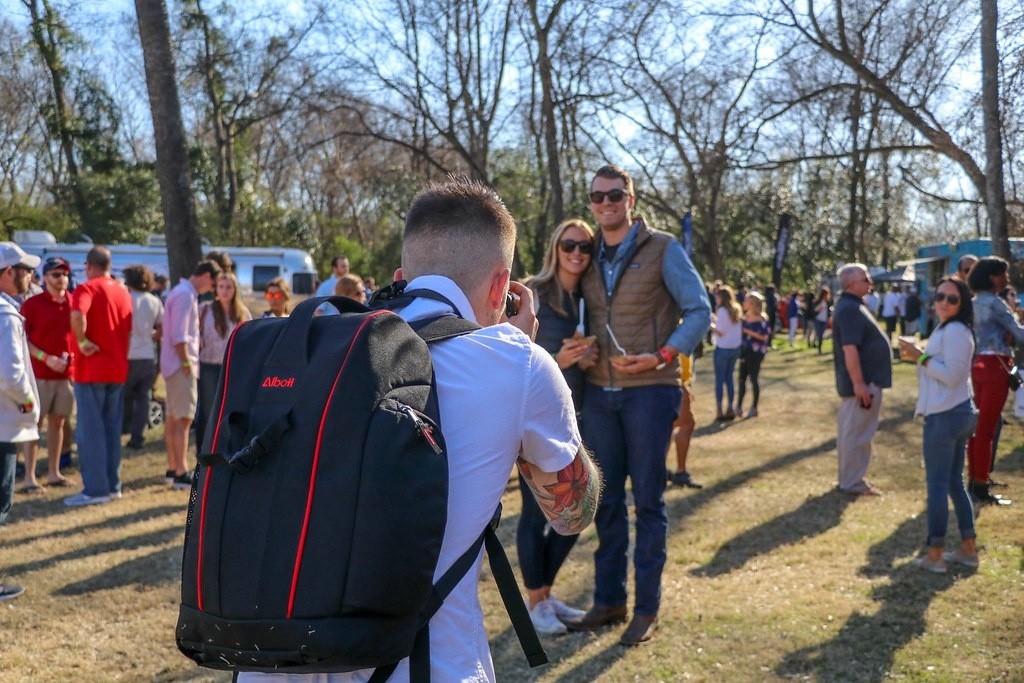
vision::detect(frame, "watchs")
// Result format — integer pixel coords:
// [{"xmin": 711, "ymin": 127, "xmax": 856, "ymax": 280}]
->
[{"xmin": 653, "ymin": 352, "xmax": 667, "ymax": 371}]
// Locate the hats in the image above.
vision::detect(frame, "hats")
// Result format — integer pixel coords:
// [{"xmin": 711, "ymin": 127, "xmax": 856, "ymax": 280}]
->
[
  {"xmin": 0, "ymin": 240, "xmax": 41, "ymax": 270},
  {"xmin": 43, "ymin": 256, "xmax": 72, "ymax": 274}
]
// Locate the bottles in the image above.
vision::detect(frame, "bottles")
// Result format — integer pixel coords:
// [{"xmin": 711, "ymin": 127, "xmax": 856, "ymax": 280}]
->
[
  {"xmin": 861, "ymin": 383, "xmax": 874, "ymax": 409},
  {"xmin": 59, "ymin": 351, "xmax": 69, "ymax": 370}
]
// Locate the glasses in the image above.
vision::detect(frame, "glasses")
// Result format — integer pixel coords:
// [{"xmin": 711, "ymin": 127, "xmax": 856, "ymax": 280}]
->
[
  {"xmin": 50, "ymin": 272, "xmax": 68, "ymax": 279},
  {"xmin": 853, "ymin": 277, "xmax": 870, "ymax": 282},
  {"xmin": 589, "ymin": 189, "xmax": 630, "ymax": 203},
  {"xmin": 353, "ymin": 287, "xmax": 367, "ymax": 296},
  {"xmin": 266, "ymin": 292, "xmax": 286, "ymax": 301},
  {"xmin": 934, "ymin": 292, "xmax": 958, "ymax": 305},
  {"xmin": 556, "ymin": 239, "xmax": 594, "ymax": 255},
  {"xmin": 15, "ymin": 265, "xmax": 35, "ymax": 274}
]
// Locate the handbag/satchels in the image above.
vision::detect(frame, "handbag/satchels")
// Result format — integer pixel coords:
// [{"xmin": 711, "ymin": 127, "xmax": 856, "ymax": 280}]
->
[{"xmin": 1007, "ymin": 365, "xmax": 1024, "ymax": 392}]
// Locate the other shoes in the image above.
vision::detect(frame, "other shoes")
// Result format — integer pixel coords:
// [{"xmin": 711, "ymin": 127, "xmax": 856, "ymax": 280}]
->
[
  {"xmin": 62, "ymin": 488, "xmax": 122, "ymax": 505},
  {"xmin": 0, "ymin": 581, "xmax": 25, "ymax": 599},
  {"xmin": 716, "ymin": 407, "xmax": 735, "ymax": 421},
  {"xmin": 667, "ymin": 469, "xmax": 702, "ymax": 488},
  {"xmin": 167, "ymin": 467, "xmax": 193, "ymax": 490},
  {"xmin": 851, "ymin": 475, "xmax": 1011, "ymax": 572},
  {"xmin": 734, "ymin": 407, "xmax": 758, "ymax": 418}
]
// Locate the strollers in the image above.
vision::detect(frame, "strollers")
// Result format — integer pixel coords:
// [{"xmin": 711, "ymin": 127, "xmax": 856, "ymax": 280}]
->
[{"xmin": 120, "ymin": 337, "xmax": 164, "ymax": 434}]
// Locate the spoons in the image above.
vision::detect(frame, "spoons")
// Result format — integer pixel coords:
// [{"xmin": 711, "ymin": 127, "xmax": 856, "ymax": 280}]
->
[
  {"xmin": 606, "ymin": 324, "xmax": 626, "ymax": 356},
  {"xmin": 576, "ymin": 297, "xmax": 584, "ymax": 336}
]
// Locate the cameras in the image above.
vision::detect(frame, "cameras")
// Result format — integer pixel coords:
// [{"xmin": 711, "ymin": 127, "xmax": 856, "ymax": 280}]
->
[{"xmin": 505, "ymin": 291, "xmax": 520, "ymax": 318}]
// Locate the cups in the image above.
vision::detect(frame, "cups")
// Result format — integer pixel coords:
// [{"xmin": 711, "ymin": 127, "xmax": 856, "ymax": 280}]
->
[{"xmin": 899, "ymin": 336, "xmax": 915, "ymax": 362}]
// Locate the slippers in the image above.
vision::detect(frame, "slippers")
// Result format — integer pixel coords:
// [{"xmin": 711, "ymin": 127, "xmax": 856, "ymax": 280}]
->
[
  {"xmin": 21, "ymin": 482, "xmax": 46, "ymax": 493},
  {"xmin": 49, "ymin": 476, "xmax": 76, "ymax": 487}
]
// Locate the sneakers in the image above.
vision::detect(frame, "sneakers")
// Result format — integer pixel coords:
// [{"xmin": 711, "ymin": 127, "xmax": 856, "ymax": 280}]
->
[
  {"xmin": 574, "ymin": 602, "xmax": 628, "ymax": 633},
  {"xmin": 547, "ymin": 596, "xmax": 584, "ymax": 622},
  {"xmin": 526, "ymin": 597, "xmax": 568, "ymax": 635},
  {"xmin": 622, "ymin": 613, "xmax": 658, "ymax": 641}
]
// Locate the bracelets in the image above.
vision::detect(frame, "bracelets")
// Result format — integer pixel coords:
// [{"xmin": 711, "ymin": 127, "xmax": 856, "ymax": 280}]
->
[
  {"xmin": 24, "ymin": 402, "xmax": 34, "ymax": 409},
  {"xmin": 184, "ymin": 362, "xmax": 192, "ymax": 368},
  {"xmin": 79, "ymin": 338, "xmax": 89, "ymax": 347},
  {"xmin": 23, "ymin": 398, "xmax": 33, "ymax": 405},
  {"xmin": 37, "ymin": 351, "xmax": 45, "ymax": 360},
  {"xmin": 919, "ymin": 353, "xmax": 930, "ymax": 365},
  {"xmin": 659, "ymin": 349, "xmax": 673, "ymax": 363},
  {"xmin": 41, "ymin": 353, "xmax": 49, "ymax": 364}
]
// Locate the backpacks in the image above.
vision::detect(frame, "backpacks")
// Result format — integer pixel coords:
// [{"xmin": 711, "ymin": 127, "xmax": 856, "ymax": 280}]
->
[{"xmin": 170, "ymin": 278, "xmax": 504, "ymax": 674}]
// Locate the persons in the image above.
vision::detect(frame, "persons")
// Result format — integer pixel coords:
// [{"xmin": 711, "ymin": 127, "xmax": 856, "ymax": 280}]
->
[
  {"xmin": 513, "ymin": 220, "xmax": 600, "ymax": 634},
  {"xmin": 0, "ymin": 241, "xmax": 382, "ymax": 602},
  {"xmin": 662, "ymin": 255, "xmax": 1024, "ymax": 504},
  {"xmin": 567, "ymin": 166, "xmax": 711, "ymax": 646},
  {"xmin": 233, "ymin": 181, "xmax": 602, "ymax": 683},
  {"xmin": 896, "ymin": 274, "xmax": 981, "ymax": 573}
]
